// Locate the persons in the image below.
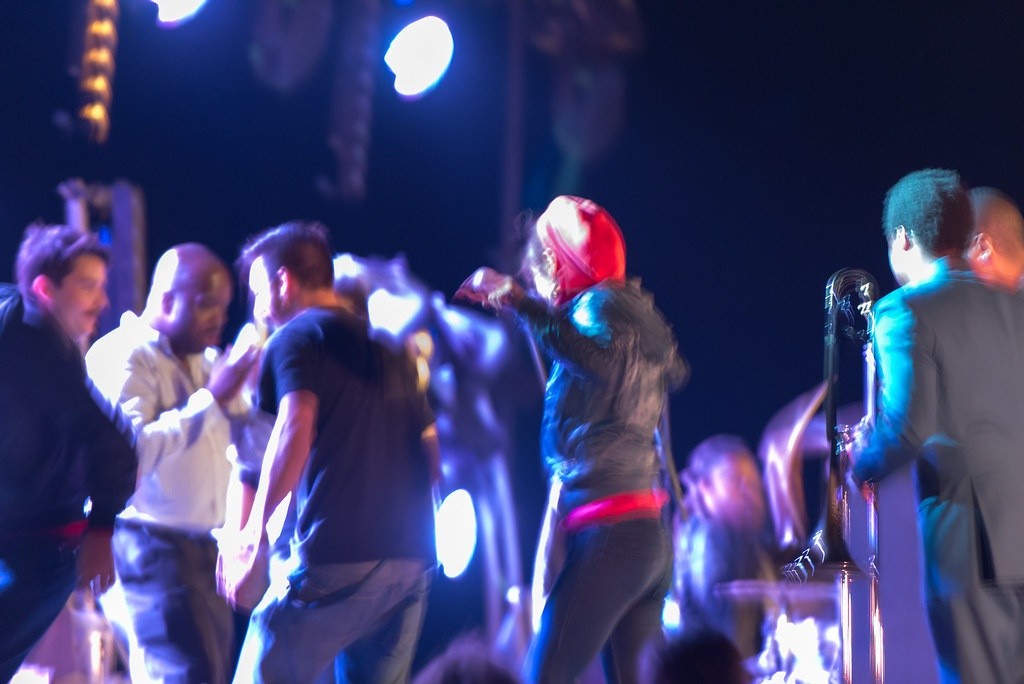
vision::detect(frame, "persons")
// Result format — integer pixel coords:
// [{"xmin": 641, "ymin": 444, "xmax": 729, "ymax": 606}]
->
[{"xmin": 0, "ymin": 168, "xmax": 1024, "ymax": 684}]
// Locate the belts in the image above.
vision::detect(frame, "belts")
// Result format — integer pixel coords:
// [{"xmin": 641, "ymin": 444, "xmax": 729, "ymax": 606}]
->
[{"xmin": 114, "ymin": 517, "xmax": 213, "ymax": 546}]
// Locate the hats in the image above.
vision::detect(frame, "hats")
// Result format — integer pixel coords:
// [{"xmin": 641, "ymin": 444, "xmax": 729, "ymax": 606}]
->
[{"xmin": 536, "ymin": 194, "xmax": 626, "ymax": 308}]
